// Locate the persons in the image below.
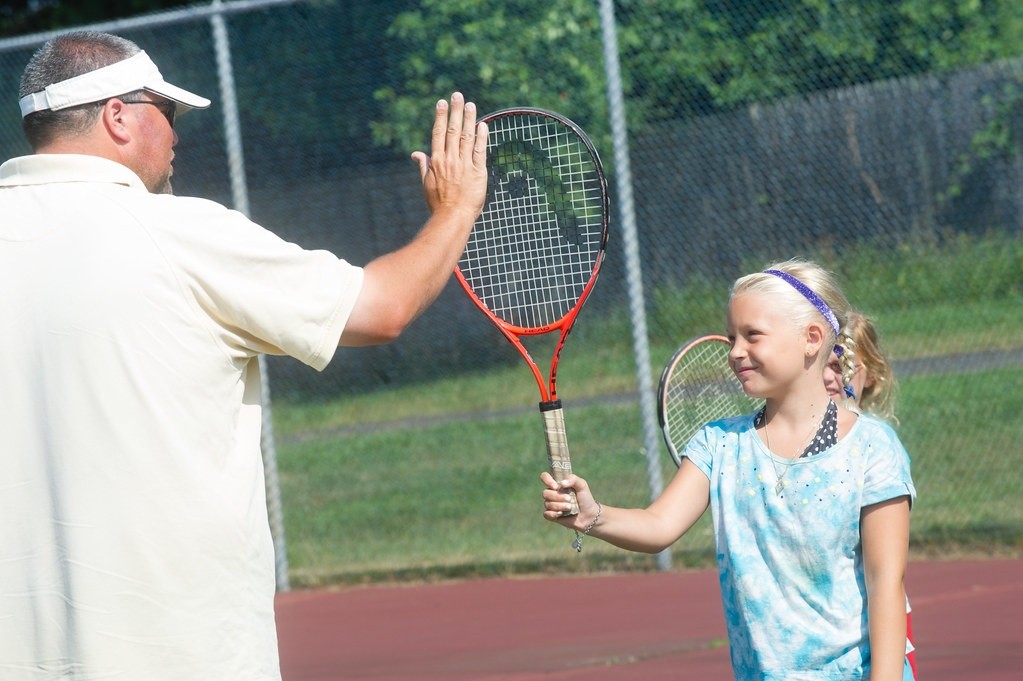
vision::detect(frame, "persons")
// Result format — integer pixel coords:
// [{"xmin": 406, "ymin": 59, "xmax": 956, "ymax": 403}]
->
[
  {"xmin": 1, "ymin": 30, "xmax": 486, "ymax": 680},
  {"xmin": 824, "ymin": 309, "xmax": 916, "ymax": 678},
  {"xmin": 541, "ymin": 260, "xmax": 918, "ymax": 681}
]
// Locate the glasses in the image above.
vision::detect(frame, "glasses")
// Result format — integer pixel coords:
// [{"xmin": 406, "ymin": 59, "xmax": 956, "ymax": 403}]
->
[{"xmin": 93, "ymin": 100, "xmax": 177, "ymax": 128}]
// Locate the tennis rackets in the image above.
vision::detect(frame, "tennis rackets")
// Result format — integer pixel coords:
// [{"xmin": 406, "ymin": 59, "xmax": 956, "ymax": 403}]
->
[
  {"xmin": 656, "ymin": 334, "xmax": 769, "ymax": 471},
  {"xmin": 451, "ymin": 105, "xmax": 613, "ymax": 519}
]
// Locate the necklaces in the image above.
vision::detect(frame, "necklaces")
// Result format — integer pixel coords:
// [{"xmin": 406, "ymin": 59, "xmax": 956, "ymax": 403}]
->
[{"xmin": 764, "ymin": 404, "xmax": 830, "ymax": 495}]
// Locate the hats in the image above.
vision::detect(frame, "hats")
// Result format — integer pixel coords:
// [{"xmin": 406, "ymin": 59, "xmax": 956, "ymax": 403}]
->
[{"xmin": 17, "ymin": 49, "xmax": 212, "ymax": 117}]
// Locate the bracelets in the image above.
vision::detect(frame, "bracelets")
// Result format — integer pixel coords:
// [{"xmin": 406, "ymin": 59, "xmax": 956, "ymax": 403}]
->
[{"xmin": 572, "ymin": 499, "xmax": 603, "ymax": 553}]
